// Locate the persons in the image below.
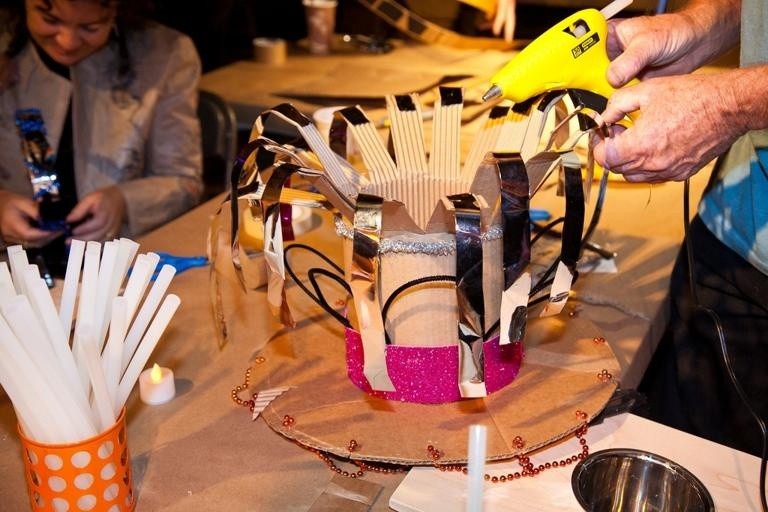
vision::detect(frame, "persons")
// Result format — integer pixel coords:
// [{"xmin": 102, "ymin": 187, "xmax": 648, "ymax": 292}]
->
[
  {"xmin": 1, "ymin": 1, "xmax": 206, "ymax": 283},
  {"xmin": 571, "ymin": 0, "xmax": 768, "ymax": 460}
]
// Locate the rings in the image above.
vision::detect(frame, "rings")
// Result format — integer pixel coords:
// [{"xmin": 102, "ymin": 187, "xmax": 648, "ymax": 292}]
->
[{"xmin": 105, "ymin": 231, "xmax": 113, "ymax": 241}]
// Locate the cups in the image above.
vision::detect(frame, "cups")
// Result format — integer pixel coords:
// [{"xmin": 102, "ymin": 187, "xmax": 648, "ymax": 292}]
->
[{"xmin": 303, "ymin": 0, "xmax": 338, "ymax": 56}]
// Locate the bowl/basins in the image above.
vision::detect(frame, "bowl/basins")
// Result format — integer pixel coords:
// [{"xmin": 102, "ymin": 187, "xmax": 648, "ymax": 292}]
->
[{"xmin": 570, "ymin": 447, "xmax": 715, "ymax": 512}]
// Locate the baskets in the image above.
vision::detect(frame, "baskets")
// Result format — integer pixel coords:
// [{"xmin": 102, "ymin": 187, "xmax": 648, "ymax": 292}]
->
[{"xmin": 16, "ymin": 403, "xmax": 136, "ymax": 512}]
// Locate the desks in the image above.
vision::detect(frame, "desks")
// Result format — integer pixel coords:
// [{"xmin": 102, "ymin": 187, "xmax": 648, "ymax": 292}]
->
[{"xmin": 0, "ymin": 32, "xmax": 739, "ymax": 511}]
